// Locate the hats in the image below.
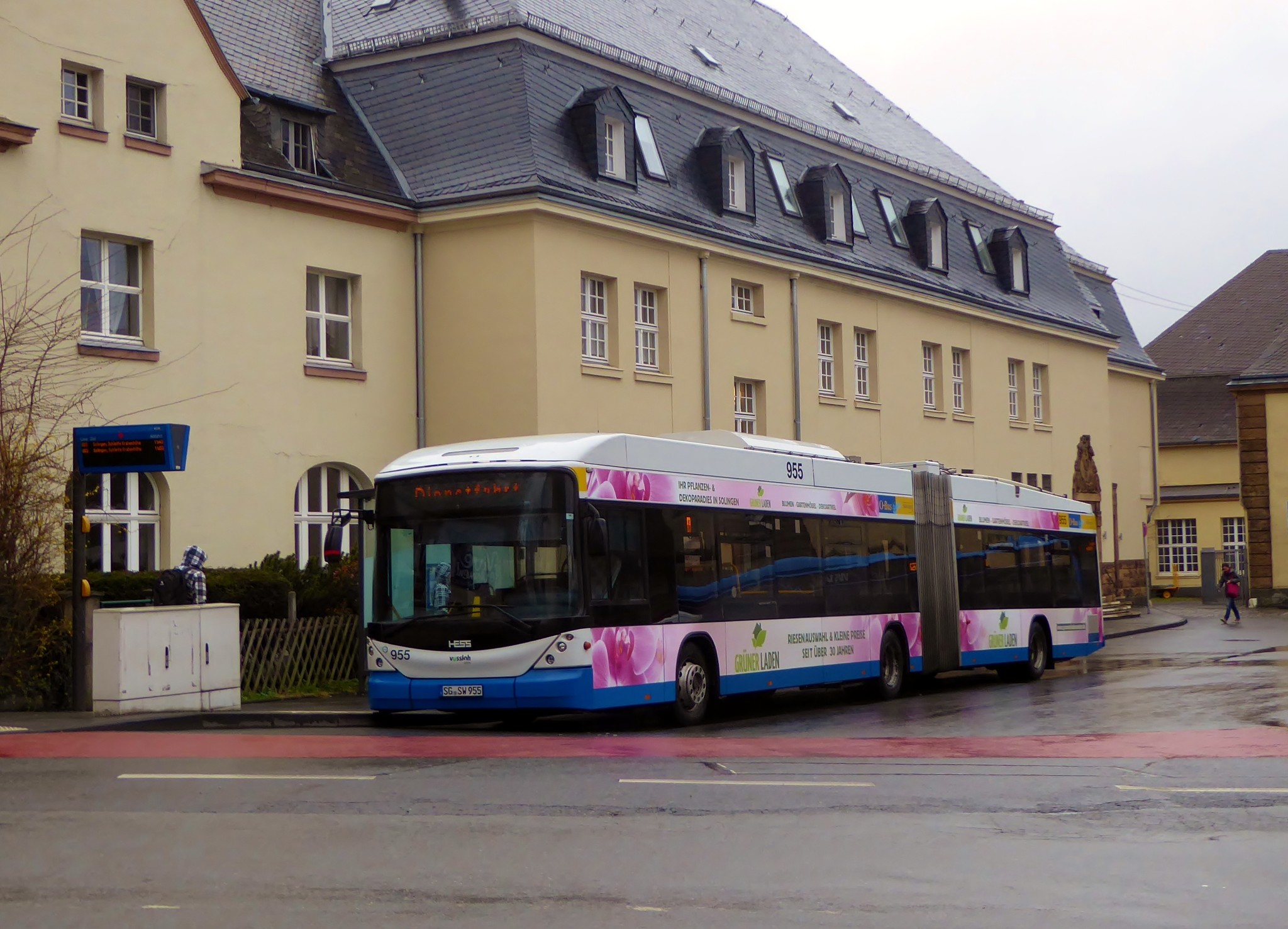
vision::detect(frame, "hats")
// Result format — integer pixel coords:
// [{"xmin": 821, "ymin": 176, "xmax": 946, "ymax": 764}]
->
[{"xmin": 1222, "ymin": 563, "xmax": 1229, "ymax": 570}]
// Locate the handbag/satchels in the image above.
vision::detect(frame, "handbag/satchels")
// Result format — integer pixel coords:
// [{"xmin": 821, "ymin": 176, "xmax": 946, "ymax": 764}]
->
[{"xmin": 1226, "ymin": 583, "xmax": 1240, "ymax": 597}]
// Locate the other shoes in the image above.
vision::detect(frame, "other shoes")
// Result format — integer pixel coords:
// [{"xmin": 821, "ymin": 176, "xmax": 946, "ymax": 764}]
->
[
  {"xmin": 1220, "ymin": 618, "xmax": 1227, "ymax": 624},
  {"xmin": 1234, "ymin": 618, "xmax": 1240, "ymax": 623}
]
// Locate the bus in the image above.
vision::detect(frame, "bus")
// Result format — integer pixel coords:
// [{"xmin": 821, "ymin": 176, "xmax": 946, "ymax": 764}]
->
[{"xmin": 324, "ymin": 429, "xmax": 1104, "ymax": 729}]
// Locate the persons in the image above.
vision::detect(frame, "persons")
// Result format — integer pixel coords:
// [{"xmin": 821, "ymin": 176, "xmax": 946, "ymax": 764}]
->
[
  {"xmin": 427, "ymin": 563, "xmax": 451, "ymax": 614},
  {"xmin": 1218, "ymin": 563, "xmax": 1241, "ymax": 624},
  {"xmin": 178, "ymin": 546, "xmax": 208, "ymax": 604}
]
